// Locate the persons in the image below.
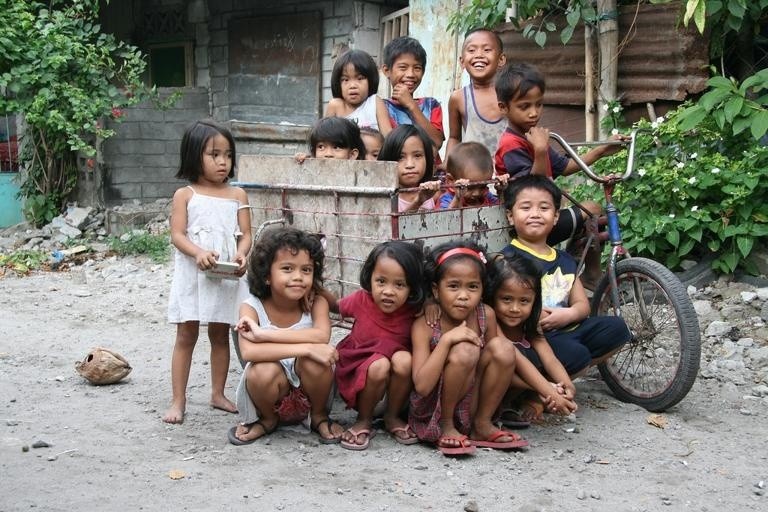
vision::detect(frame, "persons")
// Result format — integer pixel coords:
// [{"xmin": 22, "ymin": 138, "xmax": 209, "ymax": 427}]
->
[
  {"xmin": 163, "ymin": 121, "xmax": 255, "ymax": 426},
  {"xmin": 410, "ymin": 239, "xmax": 526, "ymax": 457},
  {"xmin": 299, "ymin": 240, "xmax": 442, "ymax": 452},
  {"xmin": 435, "ymin": 28, "xmax": 508, "ymax": 198},
  {"xmin": 324, "ymin": 49, "xmax": 392, "ymax": 139},
  {"xmin": 379, "ymin": 36, "xmax": 445, "ymax": 167},
  {"xmin": 297, "ymin": 117, "xmax": 510, "ymax": 213},
  {"xmin": 230, "ymin": 225, "xmax": 347, "ymax": 445},
  {"xmin": 500, "ymin": 174, "xmax": 632, "ymax": 427},
  {"xmin": 494, "ymin": 62, "xmax": 632, "ymax": 307},
  {"xmin": 484, "ymin": 249, "xmax": 578, "ymax": 430}
]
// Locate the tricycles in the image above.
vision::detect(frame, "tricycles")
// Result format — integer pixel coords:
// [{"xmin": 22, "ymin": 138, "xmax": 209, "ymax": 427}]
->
[{"xmin": 230, "ymin": 128, "xmax": 701, "ymax": 411}]
